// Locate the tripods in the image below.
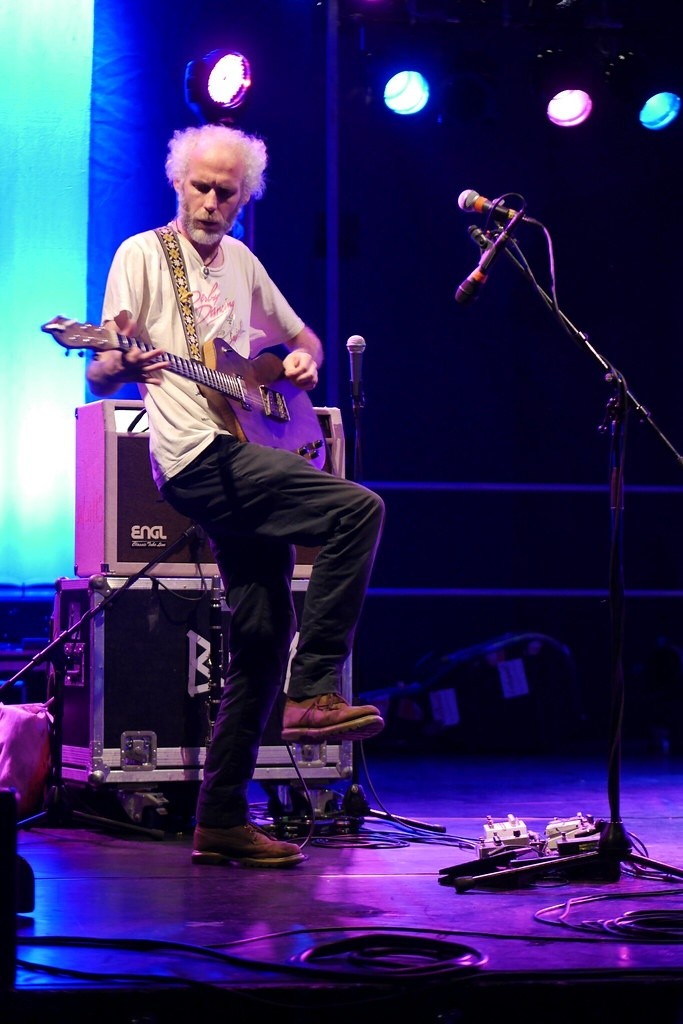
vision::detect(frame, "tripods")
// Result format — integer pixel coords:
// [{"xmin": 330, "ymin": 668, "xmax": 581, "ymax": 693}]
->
[
  {"xmin": 452, "ymin": 235, "xmax": 683, "ymax": 895},
  {"xmin": 268, "ymin": 401, "xmax": 446, "ymax": 834},
  {"xmin": 0, "ymin": 522, "xmax": 203, "ymax": 842}
]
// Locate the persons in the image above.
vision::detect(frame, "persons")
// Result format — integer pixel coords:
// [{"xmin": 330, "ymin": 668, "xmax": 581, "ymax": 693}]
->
[{"xmin": 86, "ymin": 124, "xmax": 385, "ymax": 865}]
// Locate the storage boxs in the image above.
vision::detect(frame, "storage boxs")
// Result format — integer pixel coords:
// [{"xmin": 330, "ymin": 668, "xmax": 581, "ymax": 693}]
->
[{"xmin": 46, "ymin": 576, "xmax": 354, "ymax": 785}]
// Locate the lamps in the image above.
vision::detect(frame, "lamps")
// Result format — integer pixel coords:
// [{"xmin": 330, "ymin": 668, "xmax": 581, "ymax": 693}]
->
[
  {"xmin": 601, "ymin": 47, "xmax": 681, "ymax": 130},
  {"xmin": 184, "ymin": 49, "xmax": 252, "ymax": 125}
]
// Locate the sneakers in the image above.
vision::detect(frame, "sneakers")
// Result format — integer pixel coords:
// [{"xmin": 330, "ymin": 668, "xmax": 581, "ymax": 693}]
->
[
  {"xmin": 191, "ymin": 823, "xmax": 306, "ymax": 867},
  {"xmin": 281, "ymin": 694, "xmax": 385, "ymax": 741}
]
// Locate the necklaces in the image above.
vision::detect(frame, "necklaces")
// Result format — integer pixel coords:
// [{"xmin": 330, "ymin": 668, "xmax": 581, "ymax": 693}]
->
[{"xmin": 174, "ymin": 216, "xmax": 221, "ymax": 280}]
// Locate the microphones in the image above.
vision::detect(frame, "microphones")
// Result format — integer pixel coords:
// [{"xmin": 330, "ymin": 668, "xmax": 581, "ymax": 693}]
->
[
  {"xmin": 455, "ymin": 206, "xmax": 528, "ymax": 306},
  {"xmin": 457, "ymin": 190, "xmax": 544, "ymax": 229},
  {"xmin": 345, "ymin": 334, "xmax": 366, "ymax": 419}
]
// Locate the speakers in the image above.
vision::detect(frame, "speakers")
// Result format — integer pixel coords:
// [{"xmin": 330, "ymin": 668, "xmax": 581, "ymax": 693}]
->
[{"xmin": 72, "ymin": 397, "xmax": 347, "ymax": 581}]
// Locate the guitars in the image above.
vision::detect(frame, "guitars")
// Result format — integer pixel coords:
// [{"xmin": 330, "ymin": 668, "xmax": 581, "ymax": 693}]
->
[{"xmin": 41, "ymin": 315, "xmax": 327, "ymax": 472}]
